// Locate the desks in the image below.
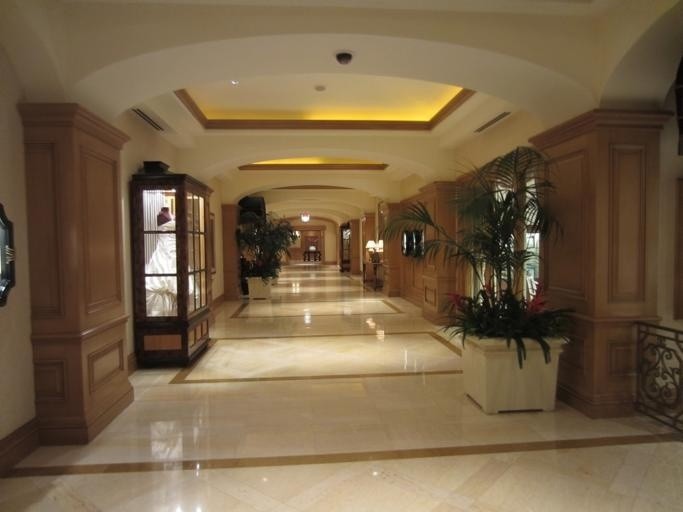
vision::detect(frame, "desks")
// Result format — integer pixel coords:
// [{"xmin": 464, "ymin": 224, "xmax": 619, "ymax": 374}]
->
[
  {"xmin": 303, "ymin": 251, "xmax": 321, "ymax": 261},
  {"xmin": 363, "ymin": 261, "xmax": 382, "ymax": 291}
]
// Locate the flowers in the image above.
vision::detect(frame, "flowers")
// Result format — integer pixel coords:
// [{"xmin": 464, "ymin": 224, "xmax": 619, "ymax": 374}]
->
[{"xmin": 432, "ymin": 275, "xmax": 584, "ymax": 369}]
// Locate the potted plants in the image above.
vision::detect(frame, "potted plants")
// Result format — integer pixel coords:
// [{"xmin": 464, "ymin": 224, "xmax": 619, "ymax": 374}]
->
[
  {"xmin": 238, "ymin": 211, "xmax": 300, "ymax": 299},
  {"xmin": 379, "ymin": 146, "xmax": 584, "ymax": 415}
]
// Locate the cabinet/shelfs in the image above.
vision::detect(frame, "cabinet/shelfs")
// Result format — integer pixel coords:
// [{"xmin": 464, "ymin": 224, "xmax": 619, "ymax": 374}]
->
[{"xmin": 128, "ymin": 173, "xmax": 214, "ymax": 368}]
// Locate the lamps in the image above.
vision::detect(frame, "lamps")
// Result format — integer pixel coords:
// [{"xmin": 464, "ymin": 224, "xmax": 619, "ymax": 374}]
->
[{"xmin": 365, "ymin": 240, "xmax": 383, "ymax": 252}]
